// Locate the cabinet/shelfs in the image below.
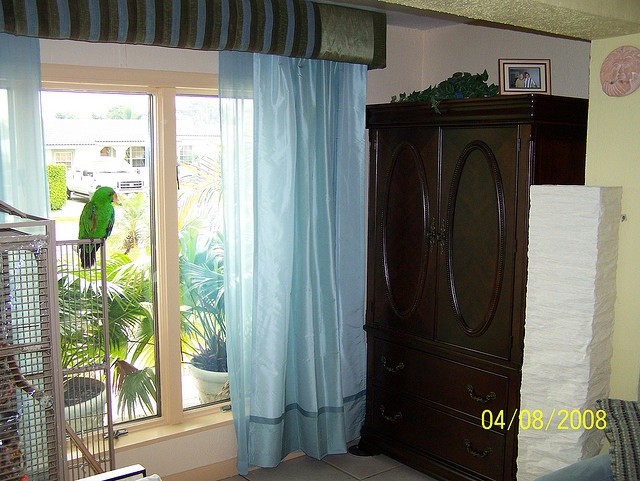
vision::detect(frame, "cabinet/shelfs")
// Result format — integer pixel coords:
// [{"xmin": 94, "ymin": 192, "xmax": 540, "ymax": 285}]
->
[{"xmin": 346, "ymin": 95, "xmax": 588, "ymax": 480}]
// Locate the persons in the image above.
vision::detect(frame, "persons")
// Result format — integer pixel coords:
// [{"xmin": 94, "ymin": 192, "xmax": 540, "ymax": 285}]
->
[{"xmin": 515, "ymin": 72, "xmax": 537, "ymax": 89}]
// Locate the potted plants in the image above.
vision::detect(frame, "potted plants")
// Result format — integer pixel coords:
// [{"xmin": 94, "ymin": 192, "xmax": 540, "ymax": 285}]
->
[
  {"xmin": 116, "ymin": 229, "xmax": 229, "ymax": 422},
  {"xmin": 56, "ymin": 255, "xmax": 153, "ymax": 433}
]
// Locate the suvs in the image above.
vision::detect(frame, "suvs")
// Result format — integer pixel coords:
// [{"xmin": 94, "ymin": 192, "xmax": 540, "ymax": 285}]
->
[{"xmin": 65, "ymin": 157, "xmax": 145, "ymax": 202}]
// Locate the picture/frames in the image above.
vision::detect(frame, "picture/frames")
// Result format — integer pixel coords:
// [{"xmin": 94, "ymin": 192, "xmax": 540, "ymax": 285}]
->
[{"xmin": 498, "ymin": 58, "xmax": 551, "ymax": 94}]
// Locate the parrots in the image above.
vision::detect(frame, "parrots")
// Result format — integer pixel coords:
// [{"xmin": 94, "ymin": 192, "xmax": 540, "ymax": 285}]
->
[{"xmin": 77, "ymin": 186, "xmax": 119, "ymax": 269}]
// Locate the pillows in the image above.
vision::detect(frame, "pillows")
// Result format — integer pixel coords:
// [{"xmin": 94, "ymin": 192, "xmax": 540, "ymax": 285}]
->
[{"xmin": 595, "ymin": 399, "xmax": 640, "ymax": 481}]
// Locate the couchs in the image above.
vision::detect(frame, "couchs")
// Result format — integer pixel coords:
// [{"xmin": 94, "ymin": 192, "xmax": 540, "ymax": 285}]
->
[{"xmin": 533, "ymin": 452, "xmax": 612, "ymax": 480}]
